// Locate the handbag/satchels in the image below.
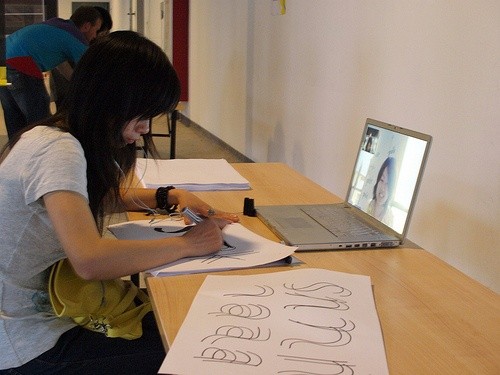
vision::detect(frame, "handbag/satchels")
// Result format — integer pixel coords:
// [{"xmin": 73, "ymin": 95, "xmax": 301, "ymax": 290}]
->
[{"xmin": 48, "ymin": 258, "xmax": 153, "ymax": 340}]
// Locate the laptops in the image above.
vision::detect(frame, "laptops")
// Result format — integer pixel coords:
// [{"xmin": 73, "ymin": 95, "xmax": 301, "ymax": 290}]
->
[{"xmin": 254, "ymin": 117, "xmax": 433, "ymax": 252}]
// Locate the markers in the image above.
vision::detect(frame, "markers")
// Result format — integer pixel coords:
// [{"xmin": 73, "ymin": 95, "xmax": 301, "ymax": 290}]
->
[{"xmin": 182, "ymin": 207, "xmax": 233, "ymax": 248}]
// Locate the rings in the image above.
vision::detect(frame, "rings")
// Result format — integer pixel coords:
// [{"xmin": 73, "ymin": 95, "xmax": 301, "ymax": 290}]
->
[{"xmin": 207, "ymin": 208, "xmax": 215, "ymax": 216}]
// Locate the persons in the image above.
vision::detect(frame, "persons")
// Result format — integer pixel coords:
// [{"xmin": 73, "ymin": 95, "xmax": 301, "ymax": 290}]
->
[
  {"xmin": 0, "ymin": 32, "xmax": 238, "ymax": 374},
  {"xmin": 366, "ymin": 157, "xmax": 398, "ymax": 228},
  {"xmin": 0, "ymin": 5, "xmax": 104, "ymax": 150},
  {"xmin": 47, "ymin": 4, "xmax": 114, "ymax": 112}
]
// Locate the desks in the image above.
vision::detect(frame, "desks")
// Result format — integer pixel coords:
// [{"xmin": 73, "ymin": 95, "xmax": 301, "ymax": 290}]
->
[{"xmin": 116, "ymin": 162, "xmax": 500, "ymax": 375}]
